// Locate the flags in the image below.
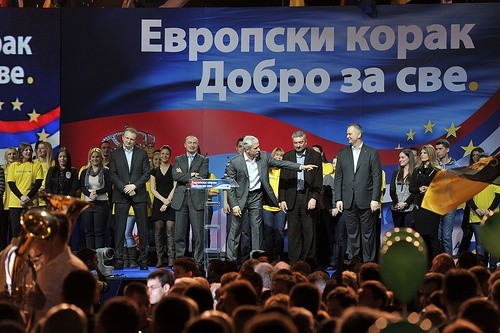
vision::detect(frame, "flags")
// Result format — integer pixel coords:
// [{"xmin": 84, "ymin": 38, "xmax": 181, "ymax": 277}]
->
[{"xmin": 420, "ymin": 147, "xmax": 500, "ymax": 216}]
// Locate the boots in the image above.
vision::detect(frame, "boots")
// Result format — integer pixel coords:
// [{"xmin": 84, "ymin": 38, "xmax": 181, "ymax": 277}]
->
[
  {"xmin": 168, "ymin": 258, "xmax": 174, "ymax": 267},
  {"xmin": 128, "ymin": 247, "xmax": 138, "ymax": 268},
  {"xmin": 156, "ymin": 258, "xmax": 163, "ymax": 267},
  {"xmin": 123, "ymin": 247, "xmax": 129, "ymax": 268}
]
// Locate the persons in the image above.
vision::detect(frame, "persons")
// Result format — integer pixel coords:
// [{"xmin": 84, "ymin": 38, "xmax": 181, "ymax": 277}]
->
[
  {"xmin": 0, "ymin": 224, "xmax": 27, "ymax": 294},
  {"xmin": 146, "ymin": 143, "xmax": 177, "ymax": 267},
  {"xmin": 170, "ymin": 136, "xmax": 209, "ymax": 268},
  {"xmin": 456, "ymin": 146, "xmax": 500, "ymax": 270},
  {"xmin": 46, "ymin": 147, "xmax": 79, "ymax": 251},
  {"xmin": 390, "ymin": 140, "xmax": 461, "ymax": 256},
  {"xmin": 112, "ymin": 202, "xmax": 138, "ymax": 268},
  {"xmin": 222, "ymin": 131, "xmax": 386, "ymax": 264},
  {"xmin": 78, "ymin": 141, "xmax": 112, "ymax": 249},
  {"xmin": 0, "ymin": 141, "xmax": 55, "ymax": 251},
  {"xmin": 21, "ymin": 211, "xmax": 87, "ymax": 333},
  {"xmin": 334, "ymin": 123, "xmax": 382, "ymax": 265},
  {"xmin": 184, "ymin": 173, "xmax": 219, "ymax": 258},
  {"xmin": 41, "ymin": 249, "xmax": 500, "ymax": 333},
  {"xmin": 109, "ymin": 128, "xmax": 151, "ymax": 269}
]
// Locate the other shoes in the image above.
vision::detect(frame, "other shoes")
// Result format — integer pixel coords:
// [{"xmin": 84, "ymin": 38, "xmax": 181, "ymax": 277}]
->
[
  {"xmin": 114, "ymin": 264, "xmax": 122, "ymax": 270},
  {"xmin": 140, "ymin": 266, "xmax": 147, "ymax": 270}
]
